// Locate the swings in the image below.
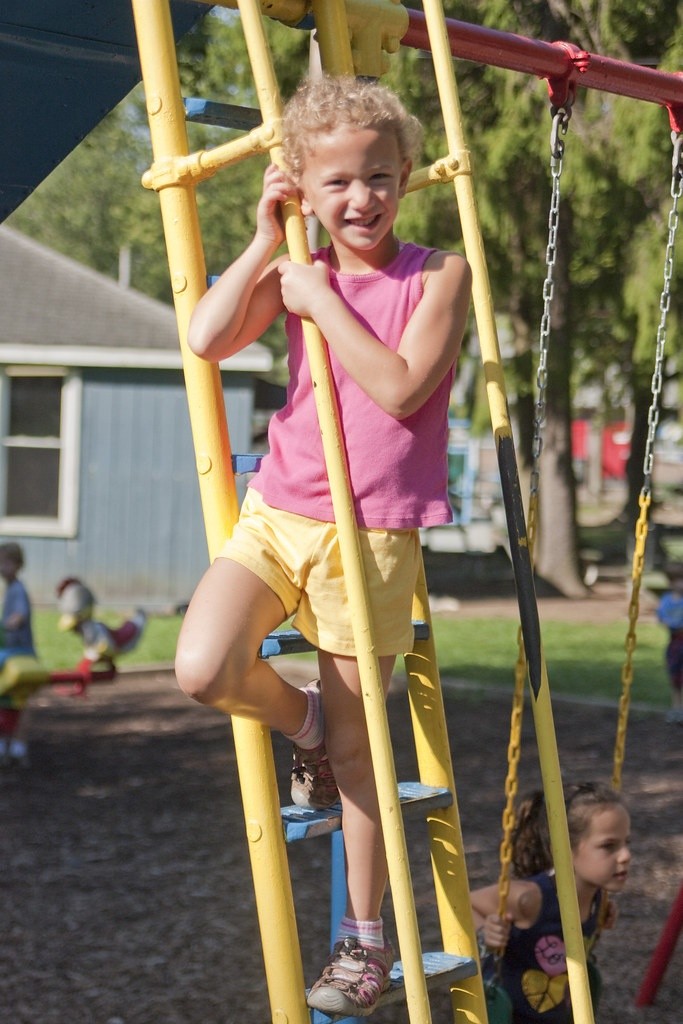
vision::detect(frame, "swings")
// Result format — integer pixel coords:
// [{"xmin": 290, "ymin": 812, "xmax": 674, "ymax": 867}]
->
[{"xmin": 480, "ymin": 101, "xmax": 682, "ymax": 1024}]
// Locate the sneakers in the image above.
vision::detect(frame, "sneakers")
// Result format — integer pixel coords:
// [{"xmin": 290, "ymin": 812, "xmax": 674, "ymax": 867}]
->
[
  {"xmin": 290, "ymin": 678, "xmax": 340, "ymax": 811},
  {"xmin": 306, "ymin": 932, "xmax": 394, "ymax": 1017}
]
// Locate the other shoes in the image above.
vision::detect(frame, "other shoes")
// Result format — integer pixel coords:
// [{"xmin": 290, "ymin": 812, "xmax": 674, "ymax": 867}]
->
[
  {"xmin": 664, "ymin": 708, "xmax": 683, "ymax": 726},
  {"xmin": 0, "ymin": 753, "xmax": 8, "ymax": 774},
  {"xmin": 8, "ymin": 753, "xmax": 31, "ymax": 774}
]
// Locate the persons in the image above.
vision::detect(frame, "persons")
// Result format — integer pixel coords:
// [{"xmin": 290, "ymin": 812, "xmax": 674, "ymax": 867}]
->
[
  {"xmin": 174, "ymin": 70, "xmax": 473, "ymax": 1015},
  {"xmin": 470, "ymin": 781, "xmax": 632, "ymax": 1024},
  {"xmin": 657, "ymin": 560, "xmax": 683, "ymax": 722},
  {"xmin": 0, "ymin": 539, "xmax": 35, "ymax": 756}
]
